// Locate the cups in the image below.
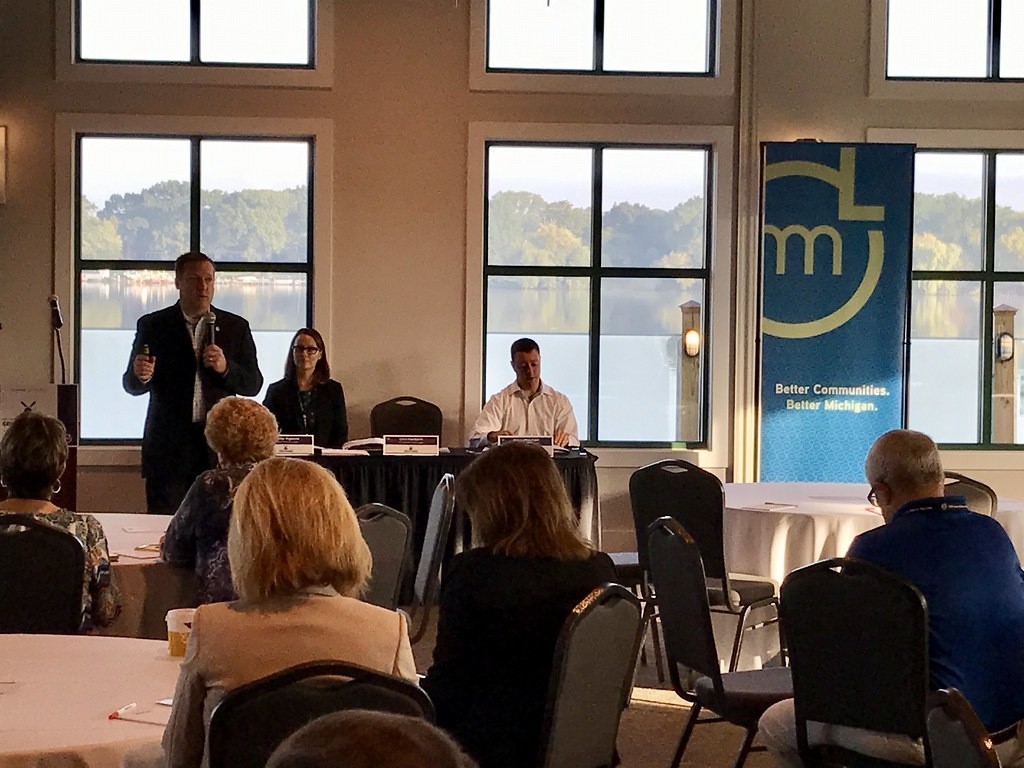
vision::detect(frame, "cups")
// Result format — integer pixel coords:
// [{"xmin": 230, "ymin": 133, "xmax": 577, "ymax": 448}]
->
[{"xmin": 165, "ymin": 607, "xmax": 197, "ymax": 660}]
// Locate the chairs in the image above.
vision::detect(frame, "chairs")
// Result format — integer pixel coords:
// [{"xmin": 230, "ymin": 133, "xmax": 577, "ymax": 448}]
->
[
  {"xmin": 0, "ymin": 511, "xmax": 86, "ymax": 635},
  {"xmin": 597, "ymin": 456, "xmax": 1004, "ymax": 767},
  {"xmin": 201, "ymin": 472, "xmax": 642, "ymax": 768},
  {"xmin": 370, "ymin": 397, "xmax": 443, "ymax": 447}
]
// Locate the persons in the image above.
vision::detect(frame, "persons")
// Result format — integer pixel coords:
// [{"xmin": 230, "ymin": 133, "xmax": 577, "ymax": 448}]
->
[
  {"xmin": 469, "ymin": 338, "xmax": 580, "ymax": 448},
  {"xmin": 262, "ymin": 327, "xmax": 350, "ymax": 449},
  {"xmin": 421, "ymin": 448, "xmax": 618, "ymax": 768},
  {"xmin": 267, "ymin": 710, "xmax": 480, "ymax": 768},
  {"xmin": 122, "ymin": 252, "xmax": 264, "ymax": 514},
  {"xmin": 162, "ymin": 456, "xmax": 419, "ymax": 768},
  {"xmin": 160, "ymin": 395, "xmax": 280, "ymax": 608},
  {"xmin": 0, "ymin": 411, "xmax": 122, "ymax": 635},
  {"xmin": 757, "ymin": 429, "xmax": 1024, "ymax": 768}
]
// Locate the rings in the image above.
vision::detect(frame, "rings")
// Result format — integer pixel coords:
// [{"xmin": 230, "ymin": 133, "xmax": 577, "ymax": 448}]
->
[{"xmin": 209, "ymin": 356, "xmax": 212, "ymax": 361}]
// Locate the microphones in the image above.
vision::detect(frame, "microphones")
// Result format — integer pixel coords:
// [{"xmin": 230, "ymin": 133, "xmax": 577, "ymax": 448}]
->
[
  {"xmin": 204, "ymin": 312, "xmax": 216, "ymax": 347},
  {"xmin": 48, "ymin": 295, "xmax": 64, "ymax": 329}
]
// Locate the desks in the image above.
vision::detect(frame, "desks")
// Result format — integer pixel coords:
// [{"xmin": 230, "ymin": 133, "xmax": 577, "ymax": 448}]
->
[
  {"xmin": 264, "ymin": 446, "xmax": 603, "ymax": 606},
  {"xmin": 723, "ymin": 484, "xmax": 888, "ymax": 682},
  {"xmin": 0, "ymin": 633, "xmax": 187, "ymax": 768},
  {"xmin": 60, "ymin": 509, "xmax": 192, "ymax": 640}
]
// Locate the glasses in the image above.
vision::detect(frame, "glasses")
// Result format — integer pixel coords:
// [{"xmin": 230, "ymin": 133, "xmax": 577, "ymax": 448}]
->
[
  {"xmin": 292, "ymin": 345, "xmax": 317, "ymax": 355},
  {"xmin": 867, "ymin": 488, "xmax": 881, "ymax": 508}
]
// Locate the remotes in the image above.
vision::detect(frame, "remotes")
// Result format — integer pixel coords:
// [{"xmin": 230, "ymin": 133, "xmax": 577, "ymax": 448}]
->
[{"xmin": 141, "ymin": 344, "xmax": 150, "ymax": 358}]
